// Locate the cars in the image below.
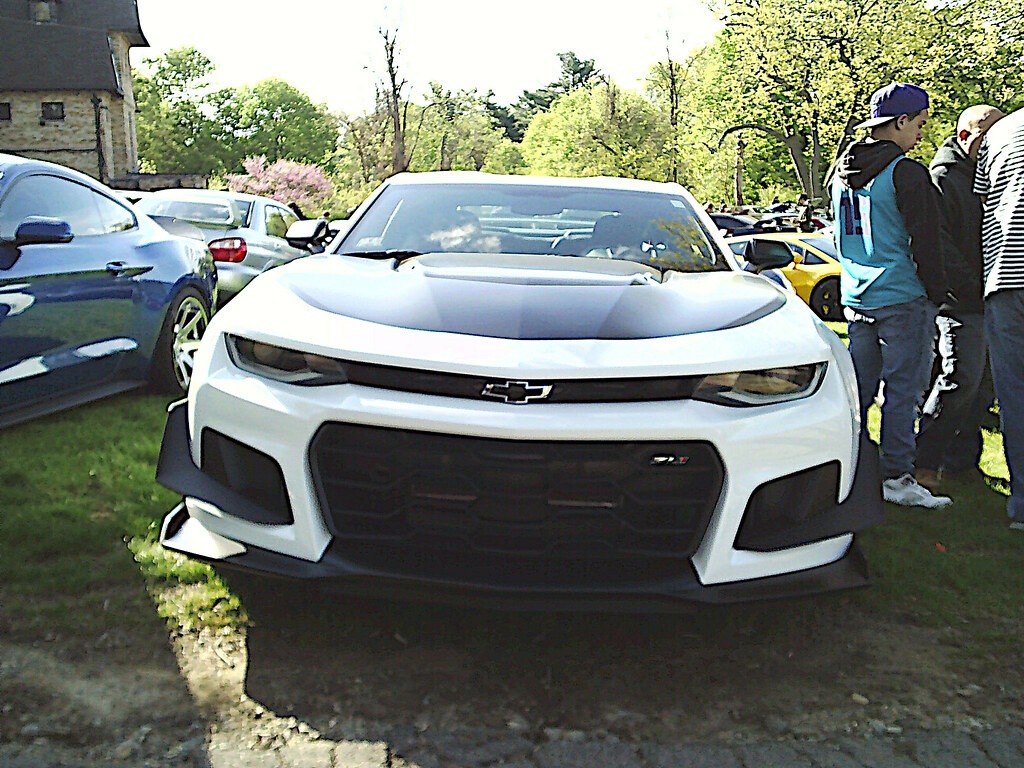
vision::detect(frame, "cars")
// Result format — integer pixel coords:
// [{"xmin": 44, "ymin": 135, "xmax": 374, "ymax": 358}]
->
[
  {"xmin": 707, "ymin": 204, "xmax": 834, "ymax": 237},
  {"xmin": 0, "ymin": 151, "xmax": 219, "ymax": 430},
  {"xmin": 132, "ymin": 188, "xmax": 326, "ymax": 311},
  {"xmin": 691, "ymin": 233, "xmax": 847, "ymax": 322},
  {"xmin": 154, "ymin": 172, "xmax": 883, "ymax": 607}
]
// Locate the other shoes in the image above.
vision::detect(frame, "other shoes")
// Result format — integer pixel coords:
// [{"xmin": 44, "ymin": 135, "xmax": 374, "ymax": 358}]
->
[
  {"xmin": 942, "ymin": 469, "xmax": 974, "ymax": 484},
  {"xmin": 884, "ymin": 474, "xmax": 953, "ymax": 511},
  {"xmin": 915, "ymin": 468, "xmax": 939, "ymax": 487}
]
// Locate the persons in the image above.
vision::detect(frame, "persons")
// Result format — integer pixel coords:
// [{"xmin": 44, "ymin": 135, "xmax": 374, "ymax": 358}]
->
[
  {"xmin": 972, "ymin": 107, "xmax": 1024, "ymax": 529},
  {"xmin": 826, "ymin": 83, "xmax": 952, "ymax": 507},
  {"xmin": 701, "ymin": 194, "xmax": 816, "ymax": 229},
  {"xmin": 910, "ymin": 104, "xmax": 1003, "ymax": 490}
]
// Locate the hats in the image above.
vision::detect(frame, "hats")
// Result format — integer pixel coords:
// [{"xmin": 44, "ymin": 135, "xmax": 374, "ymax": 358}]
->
[{"xmin": 853, "ymin": 81, "xmax": 929, "ymax": 130}]
